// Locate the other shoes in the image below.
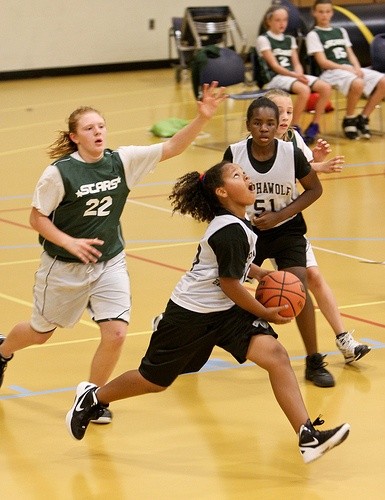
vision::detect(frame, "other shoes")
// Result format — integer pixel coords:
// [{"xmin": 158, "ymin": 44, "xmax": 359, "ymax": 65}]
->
[
  {"xmin": 0, "ymin": 333, "xmax": 13, "ymax": 388},
  {"xmin": 342, "ymin": 116, "xmax": 358, "ymax": 139},
  {"xmin": 90, "ymin": 407, "xmax": 112, "ymax": 424},
  {"xmin": 356, "ymin": 114, "xmax": 371, "ymax": 139},
  {"xmin": 303, "ymin": 123, "xmax": 319, "ymax": 145}
]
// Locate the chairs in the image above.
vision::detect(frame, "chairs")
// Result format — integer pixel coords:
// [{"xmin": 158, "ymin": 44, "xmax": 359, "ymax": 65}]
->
[{"xmin": 168, "ymin": 5, "xmax": 385, "ymax": 151}]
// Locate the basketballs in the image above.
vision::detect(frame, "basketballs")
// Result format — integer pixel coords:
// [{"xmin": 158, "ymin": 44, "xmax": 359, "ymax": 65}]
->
[{"xmin": 255, "ymin": 271, "xmax": 306, "ymax": 317}]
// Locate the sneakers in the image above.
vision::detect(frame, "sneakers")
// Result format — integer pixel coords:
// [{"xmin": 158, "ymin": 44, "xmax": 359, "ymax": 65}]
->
[
  {"xmin": 297, "ymin": 413, "xmax": 351, "ymax": 464},
  {"xmin": 304, "ymin": 353, "xmax": 335, "ymax": 387},
  {"xmin": 334, "ymin": 331, "xmax": 372, "ymax": 364},
  {"xmin": 66, "ymin": 381, "xmax": 109, "ymax": 441}
]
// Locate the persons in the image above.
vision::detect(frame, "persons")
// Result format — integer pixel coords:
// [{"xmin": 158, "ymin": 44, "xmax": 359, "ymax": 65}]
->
[
  {"xmin": 62, "ymin": 159, "xmax": 353, "ymax": 466},
  {"xmin": 0, "ymin": 79, "xmax": 233, "ymax": 426},
  {"xmin": 219, "ymin": 95, "xmax": 338, "ymax": 389},
  {"xmin": 262, "ymin": 87, "xmax": 373, "ymax": 365},
  {"xmin": 304, "ymin": 2, "xmax": 384, "ymax": 141},
  {"xmin": 254, "ymin": 5, "xmax": 333, "ymax": 143}
]
